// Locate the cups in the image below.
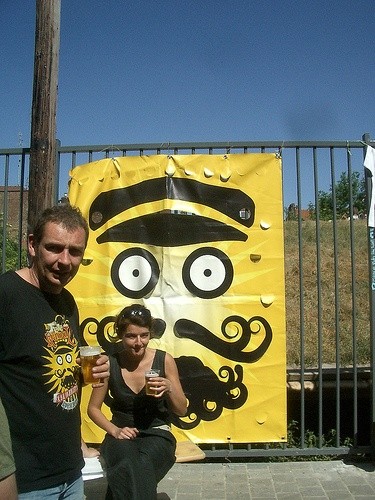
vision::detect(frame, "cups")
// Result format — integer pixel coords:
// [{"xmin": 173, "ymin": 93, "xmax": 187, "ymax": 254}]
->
[
  {"xmin": 79, "ymin": 346, "xmax": 102, "ymax": 384},
  {"xmin": 144, "ymin": 370, "xmax": 160, "ymax": 396}
]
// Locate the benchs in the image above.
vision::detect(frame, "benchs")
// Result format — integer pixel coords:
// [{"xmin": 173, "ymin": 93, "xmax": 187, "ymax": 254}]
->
[{"xmin": 81, "ymin": 440, "xmax": 206, "ymax": 500}]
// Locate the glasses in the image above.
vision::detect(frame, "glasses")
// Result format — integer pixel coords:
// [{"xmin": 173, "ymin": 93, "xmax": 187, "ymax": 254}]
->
[{"xmin": 121, "ymin": 308, "xmax": 151, "ymax": 320}]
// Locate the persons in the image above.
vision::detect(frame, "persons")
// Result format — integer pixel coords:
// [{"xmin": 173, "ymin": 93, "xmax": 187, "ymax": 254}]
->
[
  {"xmin": 0, "ymin": 206, "xmax": 111, "ymax": 500},
  {"xmin": 87, "ymin": 304, "xmax": 187, "ymax": 500},
  {"xmin": 0, "ymin": 396, "xmax": 18, "ymax": 500}
]
